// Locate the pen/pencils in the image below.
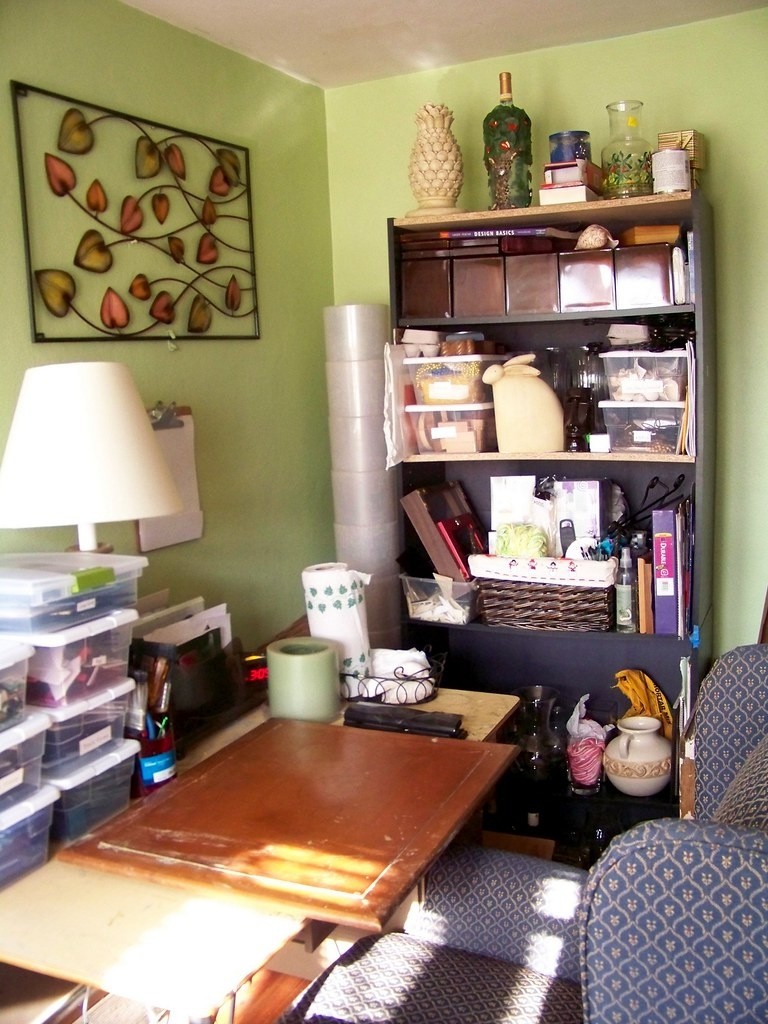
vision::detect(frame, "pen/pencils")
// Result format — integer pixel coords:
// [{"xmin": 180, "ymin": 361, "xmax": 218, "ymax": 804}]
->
[{"xmin": 125, "ymin": 658, "xmax": 172, "ymax": 738}]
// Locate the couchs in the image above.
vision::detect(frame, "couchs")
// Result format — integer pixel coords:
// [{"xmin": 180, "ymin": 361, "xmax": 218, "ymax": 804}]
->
[{"xmin": 275, "ymin": 644, "xmax": 768, "ymax": 1024}]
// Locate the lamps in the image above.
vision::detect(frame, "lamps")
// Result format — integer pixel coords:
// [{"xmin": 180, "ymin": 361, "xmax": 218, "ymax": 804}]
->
[{"xmin": 0, "ymin": 363, "xmax": 185, "ymax": 551}]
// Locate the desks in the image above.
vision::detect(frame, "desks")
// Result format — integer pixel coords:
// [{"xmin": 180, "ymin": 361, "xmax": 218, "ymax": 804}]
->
[{"xmin": 0, "ymin": 674, "xmax": 521, "ymax": 1024}]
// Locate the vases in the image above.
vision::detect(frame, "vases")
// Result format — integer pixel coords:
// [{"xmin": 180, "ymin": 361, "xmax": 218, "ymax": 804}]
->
[
  {"xmin": 600, "ymin": 100, "xmax": 655, "ymax": 199},
  {"xmin": 513, "ymin": 686, "xmax": 566, "ymax": 779}
]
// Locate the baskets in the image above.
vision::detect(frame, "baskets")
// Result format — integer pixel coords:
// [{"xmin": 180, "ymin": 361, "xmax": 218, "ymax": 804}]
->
[{"xmin": 468, "ymin": 549, "xmax": 622, "ymax": 634}]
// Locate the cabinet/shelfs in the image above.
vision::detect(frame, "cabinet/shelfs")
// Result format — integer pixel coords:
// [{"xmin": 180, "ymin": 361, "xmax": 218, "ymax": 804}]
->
[{"xmin": 386, "ymin": 187, "xmax": 717, "ymax": 863}]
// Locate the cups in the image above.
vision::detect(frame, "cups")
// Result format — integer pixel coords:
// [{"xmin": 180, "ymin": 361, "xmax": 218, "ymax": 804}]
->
[
  {"xmin": 547, "ymin": 130, "xmax": 591, "ymax": 164},
  {"xmin": 128, "ymin": 722, "xmax": 177, "ymax": 795}
]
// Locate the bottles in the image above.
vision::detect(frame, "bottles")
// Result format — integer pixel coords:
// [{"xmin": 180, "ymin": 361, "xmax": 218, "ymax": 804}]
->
[{"xmin": 616, "ymin": 548, "xmax": 638, "ymax": 634}]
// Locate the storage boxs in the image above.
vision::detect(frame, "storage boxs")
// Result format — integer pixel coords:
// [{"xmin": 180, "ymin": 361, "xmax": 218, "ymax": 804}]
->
[
  {"xmin": 0, "ymin": 551, "xmax": 149, "ymax": 892},
  {"xmin": 538, "ymin": 130, "xmax": 703, "ymax": 205},
  {"xmin": 401, "ymin": 226, "xmax": 688, "ymax": 632}
]
